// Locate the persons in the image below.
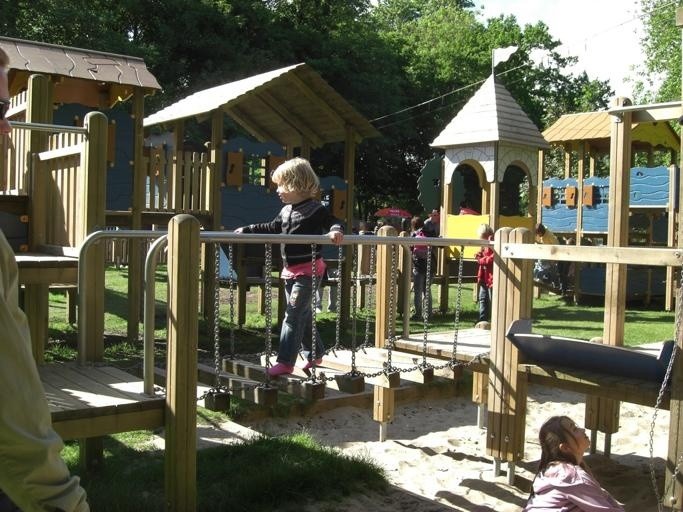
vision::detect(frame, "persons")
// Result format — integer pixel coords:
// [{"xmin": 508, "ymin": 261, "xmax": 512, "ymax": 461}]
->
[
  {"xmin": 535, "ymin": 223, "xmax": 589, "ymax": 296},
  {"xmin": 0, "ymin": 46, "xmax": 91, "ymax": 512},
  {"xmin": 234, "ymin": 158, "xmax": 345, "ymax": 377},
  {"xmin": 423, "ymin": 207, "xmax": 440, "ymax": 237},
  {"xmin": 459, "ymin": 201, "xmax": 481, "ymax": 215},
  {"xmin": 524, "ymin": 416, "xmax": 627, "ymax": 512},
  {"xmin": 410, "ymin": 218, "xmax": 437, "ymax": 321},
  {"xmin": 473, "ymin": 224, "xmax": 493, "ymax": 321}
]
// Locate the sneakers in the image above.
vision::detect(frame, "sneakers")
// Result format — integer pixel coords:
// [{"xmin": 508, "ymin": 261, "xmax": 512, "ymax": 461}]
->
[
  {"xmin": 303, "ymin": 357, "xmax": 322, "ymax": 369},
  {"xmin": 410, "ymin": 314, "xmax": 423, "ymax": 322},
  {"xmin": 267, "ymin": 363, "xmax": 293, "ymax": 376}
]
